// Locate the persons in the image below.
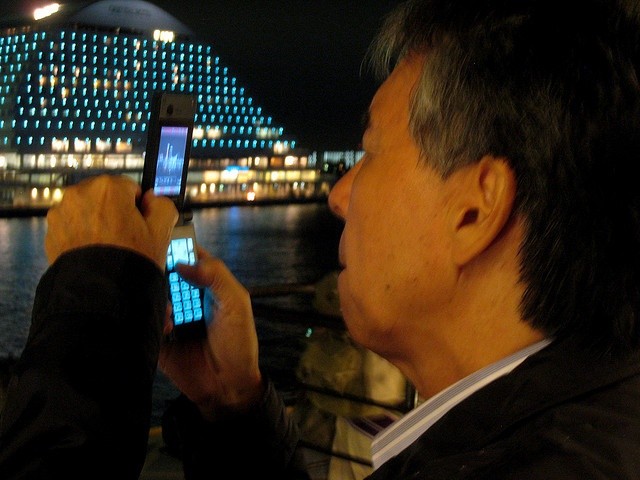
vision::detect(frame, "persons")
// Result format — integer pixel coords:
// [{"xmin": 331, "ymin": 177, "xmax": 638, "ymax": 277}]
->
[
  {"xmin": 295, "ymin": 210, "xmax": 416, "ymax": 480},
  {"xmin": 0, "ymin": 0, "xmax": 640, "ymax": 480}
]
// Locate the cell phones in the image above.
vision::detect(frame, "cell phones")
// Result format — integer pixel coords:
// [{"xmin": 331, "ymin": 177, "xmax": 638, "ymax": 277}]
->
[{"xmin": 140, "ymin": 88, "xmax": 207, "ymax": 357}]
